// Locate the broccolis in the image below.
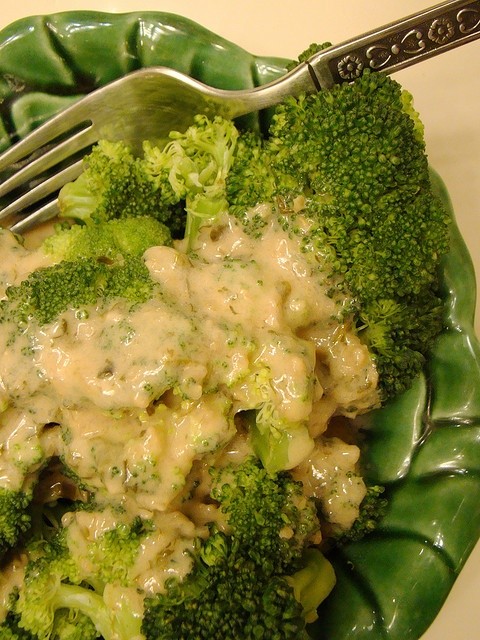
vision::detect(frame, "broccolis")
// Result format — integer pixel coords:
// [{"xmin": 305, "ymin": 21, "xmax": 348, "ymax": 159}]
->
[{"xmin": 1, "ymin": 43, "xmax": 452, "ymax": 640}]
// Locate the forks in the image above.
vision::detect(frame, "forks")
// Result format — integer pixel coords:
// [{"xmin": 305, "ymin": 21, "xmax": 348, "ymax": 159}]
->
[{"xmin": 0, "ymin": 1, "xmax": 479, "ymax": 235}]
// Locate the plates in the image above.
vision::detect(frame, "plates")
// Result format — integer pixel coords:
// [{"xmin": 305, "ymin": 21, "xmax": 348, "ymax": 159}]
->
[{"xmin": 0, "ymin": 1, "xmax": 479, "ymax": 640}]
